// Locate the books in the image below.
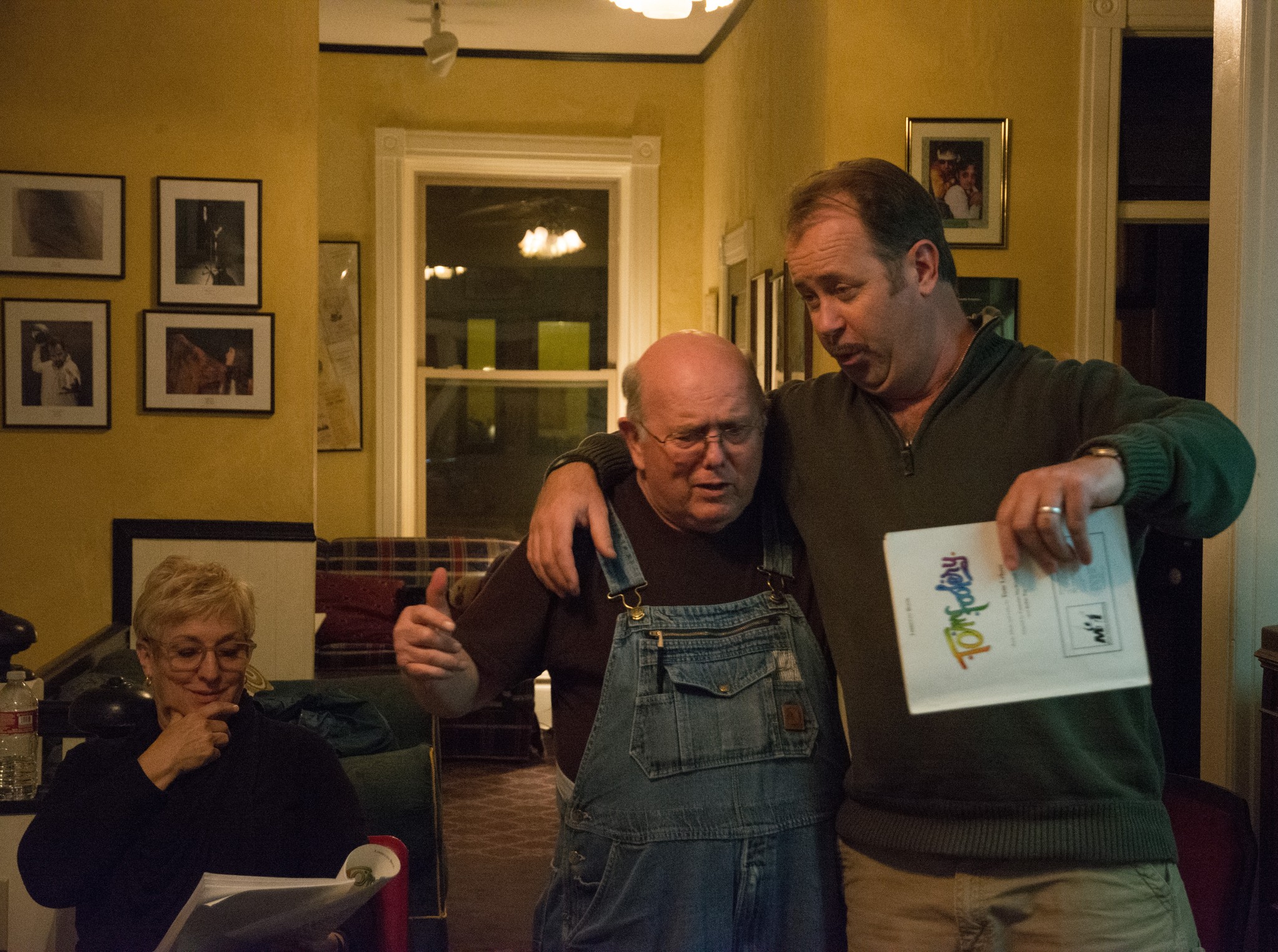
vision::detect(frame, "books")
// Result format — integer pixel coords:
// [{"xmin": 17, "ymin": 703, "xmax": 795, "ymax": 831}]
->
[
  {"xmin": 154, "ymin": 839, "xmax": 401, "ymax": 951},
  {"xmin": 882, "ymin": 504, "xmax": 1152, "ymax": 714}
]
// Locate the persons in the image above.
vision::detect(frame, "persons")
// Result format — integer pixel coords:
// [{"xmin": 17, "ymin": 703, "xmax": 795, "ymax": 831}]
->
[
  {"xmin": 16, "ymin": 553, "xmax": 393, "ymax": 951},
  {"xmin": 207, "ymin": 223, "xmax": 229, "ymax": 273},
  {"xmin": 391, "ymin": 329, "xmax": 854, "ymax": 952},
  {"xmin": 176, "ymin": 336, "xmax": 252, "ymax": 395},
  {"xmin": 523, "ymin": 157, "xmax": 1256, "ymax": 951},
  {"xmin": 928, "ymin": 144, "xmax": 982, "ymax": 219},
  {"xmin": 30, "ymin": 338, "xmax": 84, "ymax": 407}
]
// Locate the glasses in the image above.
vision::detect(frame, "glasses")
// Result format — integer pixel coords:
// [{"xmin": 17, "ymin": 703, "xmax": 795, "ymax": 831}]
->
[
  {"xmin": 635, "ymin": 409, "xmax": 769, "ymax": 459},
  {"xmin": 144, "ymin": 637, "xmax": 257, "ymax": 673}
]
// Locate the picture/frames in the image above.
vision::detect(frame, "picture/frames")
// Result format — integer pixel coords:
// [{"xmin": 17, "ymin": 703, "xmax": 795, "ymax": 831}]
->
[
  {"xmin": 783, "ymin": 259, "xmax": 813, "ymax": 385},
  {"xmin": 905, "ymin": 115, "xmax": 1007, "ymax": 250},
  {"xmin": 141, "ymin": 308, "xmax": 274, "ymax": 413},
  {"xmin": 768, "ymin": 269, "xmax": 785, "ymax": 393},
  {"xmin": 748, "ymin": 270, "xmax": 767, "ymax": 396},
  {"xmin": 317, "ymin": 239, "xmax": 363, "ymax": 452},
  {"xmin": 0, "ymin": 169, "xmax": 126, "ymax": 281},
  {"xmin": 156, "ymin": 174, "xmax": 262, "ymax": 308},
  {"xmin": 0, "ymin": 296, "xmax": 112, "ymax": 429},
  {"xmin": 956, "ymin": 277, "xmax": 1019, "ymax": 343}
]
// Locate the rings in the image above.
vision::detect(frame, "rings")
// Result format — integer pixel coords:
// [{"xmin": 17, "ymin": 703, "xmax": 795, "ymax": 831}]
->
[{"xmin": 1036, "ymin": 505, "xmax": 1063, "ymax": 515}]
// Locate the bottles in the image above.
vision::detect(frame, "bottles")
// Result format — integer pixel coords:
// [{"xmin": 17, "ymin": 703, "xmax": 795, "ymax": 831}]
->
[{"xmin": 0, "ymin": 671, "xmax": 38, "ymax": 801}]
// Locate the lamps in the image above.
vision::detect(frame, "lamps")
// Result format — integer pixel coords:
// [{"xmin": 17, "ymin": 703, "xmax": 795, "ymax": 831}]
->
[
  {"xmin": 420, "ymin": 0, "xmax": 459, "ymax": 80},
  {"xmin": 517, "ymin": 217, "xmax": 587, "ymax": 262}
]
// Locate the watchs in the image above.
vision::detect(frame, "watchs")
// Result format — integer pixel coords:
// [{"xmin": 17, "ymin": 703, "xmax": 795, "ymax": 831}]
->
[{"xmin": 1089, "ymin": 445, "xmax": 1123, "ymax": 464}]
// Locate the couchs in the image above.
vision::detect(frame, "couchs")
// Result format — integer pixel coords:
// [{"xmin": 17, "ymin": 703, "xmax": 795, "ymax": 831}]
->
[
  {"xmin": 316, "ymin": 535, "xmax": 544, "ymax": 765},
  {"xmin": 36, "ymin": 646, "xmax": 452, "ymax": 925}
]
